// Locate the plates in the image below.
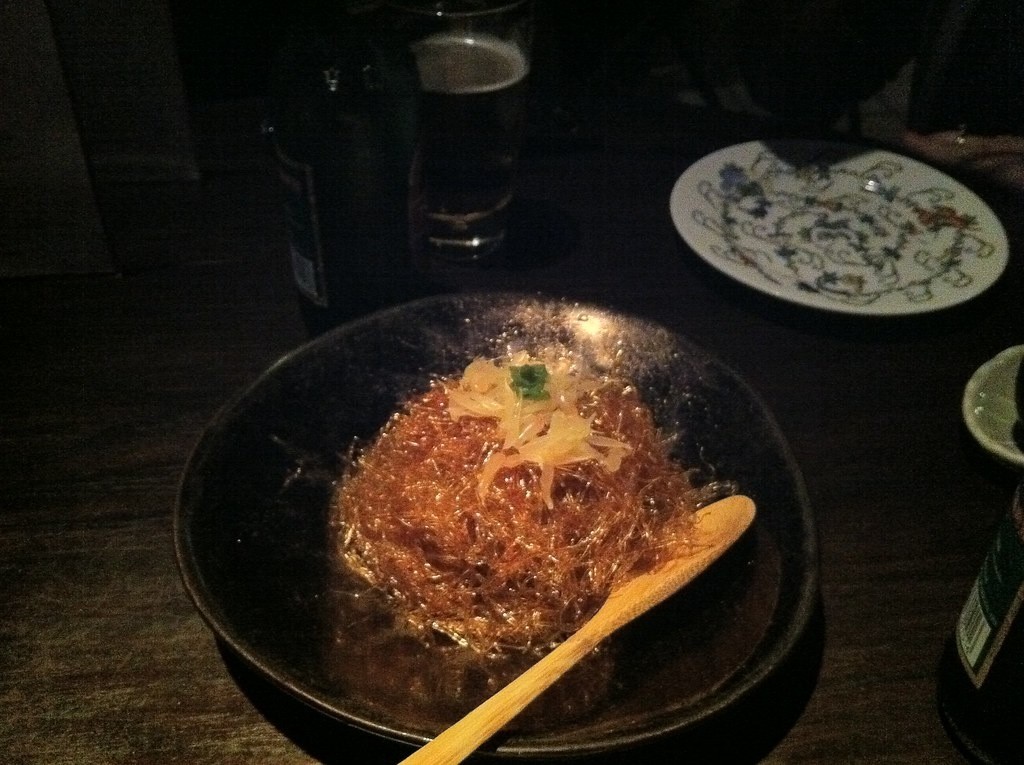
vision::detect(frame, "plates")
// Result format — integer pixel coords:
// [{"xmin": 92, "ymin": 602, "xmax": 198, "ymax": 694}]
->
[
  {"xmin": 172, "ymin": 290, "xmax": 820, "ymax": 754},
  {"xmin": 669, "ymin": 137, "xmax": 1008, "ymax": 315},
  {"xmin": 962, "ymin": 345, "xmax": 1024, "ymax": 467}
]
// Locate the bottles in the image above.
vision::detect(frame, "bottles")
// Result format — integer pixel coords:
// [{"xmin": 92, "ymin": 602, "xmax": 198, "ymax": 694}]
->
[
  {"xmin": 942, "ymin": 479, "xmax": 1024, "ymax": 704},
  {"xmin": 906, "ymin": 0, "xmax": 1024, "ymax": 181},
  {"xmin": 270, "ymin": 1, "xmax": 431, "ymax": 326}
]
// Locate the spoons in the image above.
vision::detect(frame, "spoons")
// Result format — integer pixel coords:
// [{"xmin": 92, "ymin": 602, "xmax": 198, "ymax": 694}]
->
[{"xmin": 397, "ymin": 496, "xmax": 755, "ymax": 765}]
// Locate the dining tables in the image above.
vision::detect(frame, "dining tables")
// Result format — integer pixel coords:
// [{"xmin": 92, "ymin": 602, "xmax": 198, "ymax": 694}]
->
[{"xmin": 0, "ymin": 129, "xmax": 1024, "ymax": 762}]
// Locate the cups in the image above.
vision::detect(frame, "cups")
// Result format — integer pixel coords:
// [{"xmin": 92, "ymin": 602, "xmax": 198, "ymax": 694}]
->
[{"xmin": 415, "ymin": 1, "xmax": 530, "ymax": 261}]
